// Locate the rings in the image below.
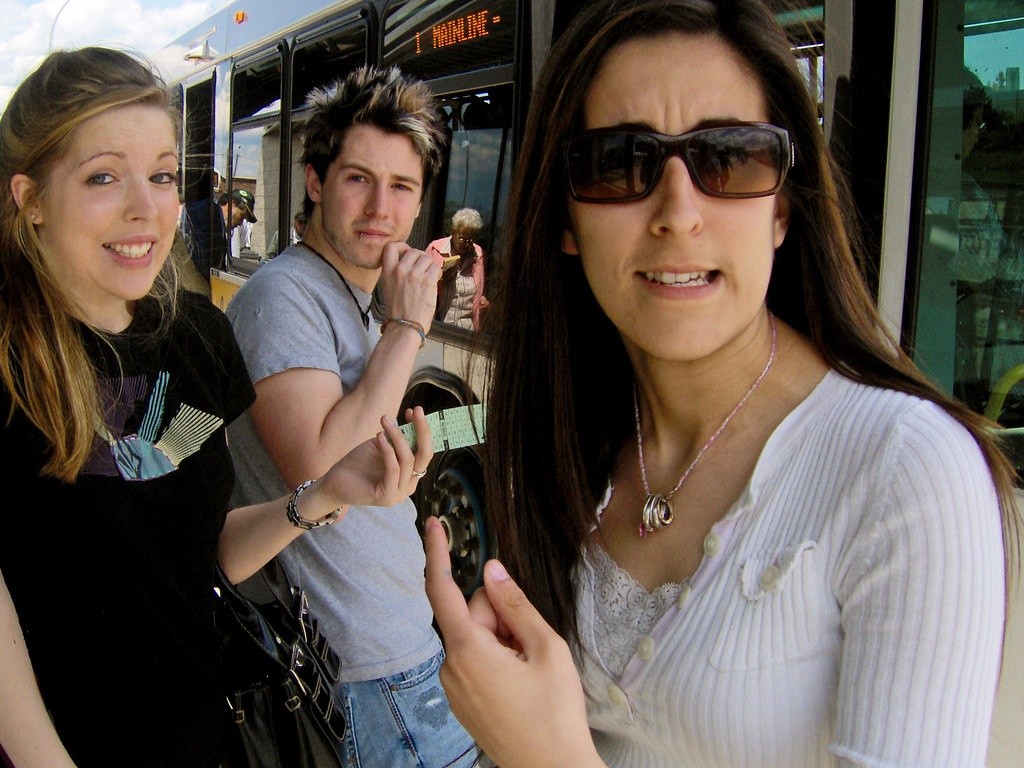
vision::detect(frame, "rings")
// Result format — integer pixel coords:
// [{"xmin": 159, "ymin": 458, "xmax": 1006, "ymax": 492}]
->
[{"xmin": 413, "ymin": 470, "xmax": 427, "ymax": 478}]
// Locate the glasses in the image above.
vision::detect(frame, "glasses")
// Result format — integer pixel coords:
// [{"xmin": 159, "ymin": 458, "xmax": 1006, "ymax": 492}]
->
[
  {"xmin": 563, "ymin": 121, "xmax": 796, "ymax": 204},
  {"xmin": 458, "ymin": 231, "xmax": 473, "ymax": 245}
]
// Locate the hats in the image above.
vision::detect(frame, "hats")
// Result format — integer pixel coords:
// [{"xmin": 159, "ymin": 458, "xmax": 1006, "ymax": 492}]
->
[
  {"xmin": 295, "ymin": 213, "xmax": 307, "ymax": 223},
  {"xmin": 224, "ymin": 188, "xmax": 257, "ymax": 223}
]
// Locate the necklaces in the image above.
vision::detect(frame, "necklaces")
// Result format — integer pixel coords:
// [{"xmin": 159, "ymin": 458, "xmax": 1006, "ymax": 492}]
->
[
  {"xmin": 297, "ymin": 240, "xmax": 373, "ymax": 331},
  {"xmin": 632, "ymin": 310, "xmax": 777, "ymax": 538}
]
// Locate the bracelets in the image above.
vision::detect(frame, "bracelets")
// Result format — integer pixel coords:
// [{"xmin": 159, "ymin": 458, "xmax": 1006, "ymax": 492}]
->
[
  {"xmin": 380, "ymin": 316, "xmax": 426, "ymax": 351},
  {"xmin": 286, "ymin": 480, "xmax": 343, "ymax": 529}
]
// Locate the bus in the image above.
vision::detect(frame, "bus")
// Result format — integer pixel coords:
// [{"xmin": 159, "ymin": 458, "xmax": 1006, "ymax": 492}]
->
[{"xmin": 117, "ymin": 0, "xmax": 1024, "ymax": 628}]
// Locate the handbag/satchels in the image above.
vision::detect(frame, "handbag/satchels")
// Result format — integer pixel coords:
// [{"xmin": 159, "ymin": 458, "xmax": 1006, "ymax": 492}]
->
[{"xmin": 209, "ymin": 530, "xmax": 347, "ymax": 768}]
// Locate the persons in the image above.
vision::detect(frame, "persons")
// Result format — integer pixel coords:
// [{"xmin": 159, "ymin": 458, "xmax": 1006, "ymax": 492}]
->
[
  {"xmin": 267, "ymin": 212, "xmax": 306, "ymax": 258},
  {"xmin": 961, "ymin": 65, "xmax": 1016, "ymax": 261},
  {"xmin": 425, "ymin": 207, "xmax": 484, "ymax": 333},
  {"xmin": 212, "ymin": 188, "xmax": 257, "ymax": 271},
  {"xmin": 0, "ymin": 46, "xmax": 433, "ymax": 767},
  {"xmin": 222, "ymin": 68, "xmax": 491, "ymax": 768},
  {"xmin": 423, "ymin": 2, "xmax": 1022, "ymax": 768}
]
